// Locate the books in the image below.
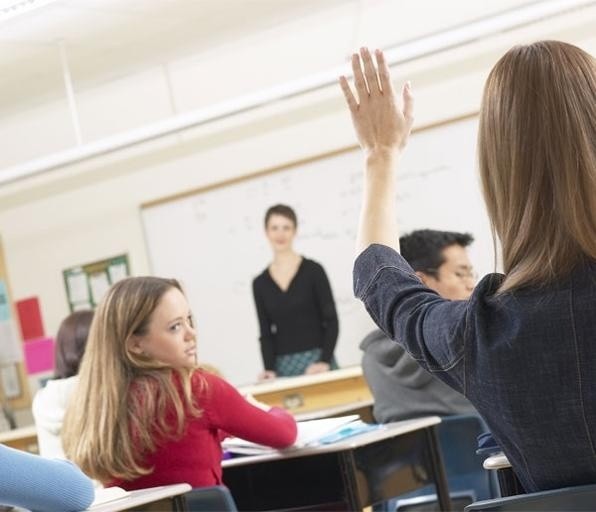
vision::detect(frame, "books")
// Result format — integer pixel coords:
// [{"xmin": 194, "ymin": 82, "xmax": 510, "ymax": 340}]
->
[{"xmin": 221, "ymin": 414, "xmax": 371, "ymax": 461}]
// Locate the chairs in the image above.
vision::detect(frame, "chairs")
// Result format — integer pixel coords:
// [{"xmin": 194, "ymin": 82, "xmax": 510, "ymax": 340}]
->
[
  {"xmin": 462, "ymin": 484, "xmax": 596, "ymax": 512},
  {"xmin": 374, "ymin": 413, "xmax": 501, "ymax": 505}
]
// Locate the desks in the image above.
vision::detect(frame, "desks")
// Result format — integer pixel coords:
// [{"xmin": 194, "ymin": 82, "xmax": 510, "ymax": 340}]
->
[
  {"xmin": 224, "ymin": 415, "xmax": 452, "ymax": 511},
  {"xmin": 87, "ymin": 482, "xmax": 193, "ymax": 512},
  {"xmin": 239, "ymin": 364, "xmax": 375, "ymax": 422}
]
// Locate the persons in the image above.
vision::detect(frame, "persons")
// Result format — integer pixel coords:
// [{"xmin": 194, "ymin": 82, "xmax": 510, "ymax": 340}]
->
[
  {"xmin": 33, "ymin": 311, "xmax": 97, "ymax": 465},
  {"xmin": 360, "ymin": 229, "xmax": 479, "ymax": 431},
  {"xmin": 338, "ymin": 42, "xmax": 595, "ymax": 492},
  {"xmin": 64, "ymin": 278, "xmax": 296, "ymax": 491},
  {"xmin": 253, "ymin": 205, "xmax": 342, "ymax": 380},
  {"xmin": 0, "ymin": 445, "xmax": 95, "ymax": 511}
]
